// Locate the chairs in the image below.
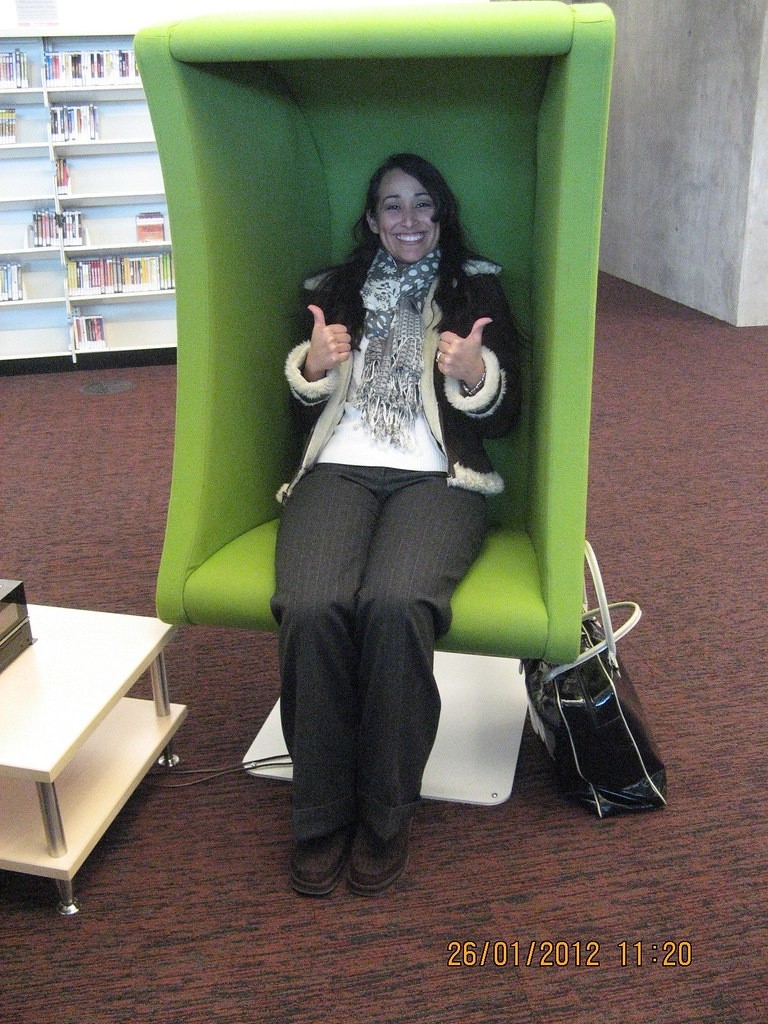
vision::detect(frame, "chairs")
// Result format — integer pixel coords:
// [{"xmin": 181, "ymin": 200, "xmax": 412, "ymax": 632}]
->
[{"xmin": 132, "ymin": 0, "xmax": 616, "ymax": 806}]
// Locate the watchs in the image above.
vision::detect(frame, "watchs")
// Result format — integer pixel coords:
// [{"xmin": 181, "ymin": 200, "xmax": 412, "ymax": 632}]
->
[{"xmin": 461, "ymin": 362, "xmax": 486, "ymax": 396}]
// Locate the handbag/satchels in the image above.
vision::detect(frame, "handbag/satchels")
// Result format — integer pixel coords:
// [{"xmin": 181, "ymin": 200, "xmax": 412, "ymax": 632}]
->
[{"xmin": 518, "ymin": 541, "xmax": 667, "ymax": 818}]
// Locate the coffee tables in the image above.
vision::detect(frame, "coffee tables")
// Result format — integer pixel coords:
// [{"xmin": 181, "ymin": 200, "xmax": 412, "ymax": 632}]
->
[{"xmin": 0, "ymin": 604, "xmax": 189, "ymax": 915}]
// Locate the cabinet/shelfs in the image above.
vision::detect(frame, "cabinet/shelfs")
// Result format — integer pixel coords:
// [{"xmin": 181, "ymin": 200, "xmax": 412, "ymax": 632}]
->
[{"xmin": 0, "ymin": 31, "xmax": 177, "ymax": 378}]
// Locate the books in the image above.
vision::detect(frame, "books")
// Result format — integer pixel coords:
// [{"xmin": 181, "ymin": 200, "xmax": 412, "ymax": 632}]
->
[
  {"xmin": 0, "ymin": 109, "xmax": 16, "ymax": 144},
  {"xmin": 67, "ymin": 250, "xmax": 175, "ymax": 296},
  {"xmin": 46, "ymin": 49, "xmax": 142, "ymax": 88},
  {"xmin": 0, "ymin": 261, "xmax": 23, "ymax": 301},
  {"xmin": 61, "ymin": 211, "xmax": 83, "ymax": 246},
  {"xmin": 28, "ymin": 209, "xmax": 57, "ymax": 247},
  {"xmin": 74, "ymin": 316, "xmax": 105, "ymax": 350},
  {"xmin": 50, "ymin": 104, "xmax": 98, "ymax": 141},
  {"xmin": 0, "ymin": 49, "xmax": 28, "ymax": 88},
  {"xmin": 56, "ymin": 158, "xmax": 69, "ymax": 195}
]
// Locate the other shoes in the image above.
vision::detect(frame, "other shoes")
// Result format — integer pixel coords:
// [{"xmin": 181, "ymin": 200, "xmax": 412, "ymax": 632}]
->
[
  {"xmin": 345, "ymin": 822, "xmax": 410, "ymax": 896},
  {"xmin": 289, "ymin": 822, "xmax": 350, "ymax": 894}
]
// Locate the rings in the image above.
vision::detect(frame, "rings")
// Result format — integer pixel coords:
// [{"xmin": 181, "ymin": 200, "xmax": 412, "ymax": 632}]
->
[{"xmin": 436, "ymin": 352, "xmax": 444, "ymax": 363}]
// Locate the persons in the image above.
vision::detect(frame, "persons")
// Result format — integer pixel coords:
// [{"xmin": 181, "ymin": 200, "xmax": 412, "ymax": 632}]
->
[{"xmin": 270, "ymin": 153, "xmax": 522, "ymax": 894}]
